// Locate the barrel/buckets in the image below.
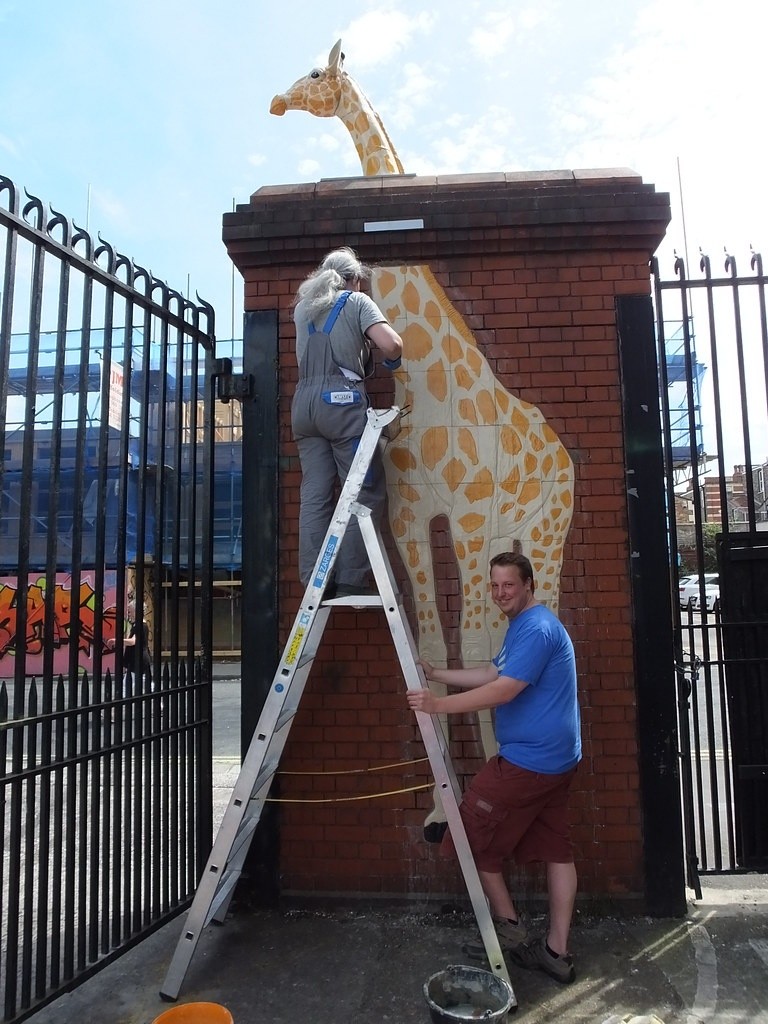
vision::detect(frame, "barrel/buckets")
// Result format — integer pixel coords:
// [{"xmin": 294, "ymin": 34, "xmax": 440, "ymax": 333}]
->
[
  {"xmin": 424, "ymin": 965, "xmax": 518, "ymax": 1024},
  {"xmin": 152, "ymin": 1002, "xmax": 233, "ymax": 1024}
]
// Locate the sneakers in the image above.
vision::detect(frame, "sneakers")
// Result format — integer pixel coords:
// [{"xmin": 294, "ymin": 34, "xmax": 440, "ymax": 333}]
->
[
  {"xmin": 462, "ymin": 917, "xmax": 532, "ymax": 958},
  {"xmin": 511, "ymin": 937, "xmax": 573, "ymax": 983}
]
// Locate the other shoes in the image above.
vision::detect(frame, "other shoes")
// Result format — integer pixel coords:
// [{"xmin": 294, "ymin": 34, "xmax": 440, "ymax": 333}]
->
[{"xmin": 336, "ymin": 582, "xmax": 380, "ymax": 597}]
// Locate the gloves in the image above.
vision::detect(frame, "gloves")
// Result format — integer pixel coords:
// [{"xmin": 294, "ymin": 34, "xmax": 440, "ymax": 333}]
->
[{"xmin": 382, "ymin": 356, "xmax": 402, "ymax": 371}]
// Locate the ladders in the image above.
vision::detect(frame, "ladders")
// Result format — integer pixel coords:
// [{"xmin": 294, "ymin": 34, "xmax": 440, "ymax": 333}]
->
[{"xmin": 158, "ymin": 405, "xmax": 522, "ymax": 1014}]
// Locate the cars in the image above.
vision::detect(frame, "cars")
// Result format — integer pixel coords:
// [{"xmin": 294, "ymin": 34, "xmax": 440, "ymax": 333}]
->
[
  {"xmin": 679, "ymin": 571, "xmax": 719, "ymax": 611},
  {"xmin": 690, "ymin": 587, "xmax": 721, "ymax": 612}
]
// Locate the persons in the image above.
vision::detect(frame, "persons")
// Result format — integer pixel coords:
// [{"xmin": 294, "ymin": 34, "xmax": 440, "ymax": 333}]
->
[
  {"xmin": 291, "ymin": 247, "xmax": 404, "ymax": 609},
  {"xmin": 106, "ymin": 600, "xmax": 167, "ymax": 723},
  {"xmin": 406, "ymin": 552, "xmax": 583, "ymax": 986}
]
